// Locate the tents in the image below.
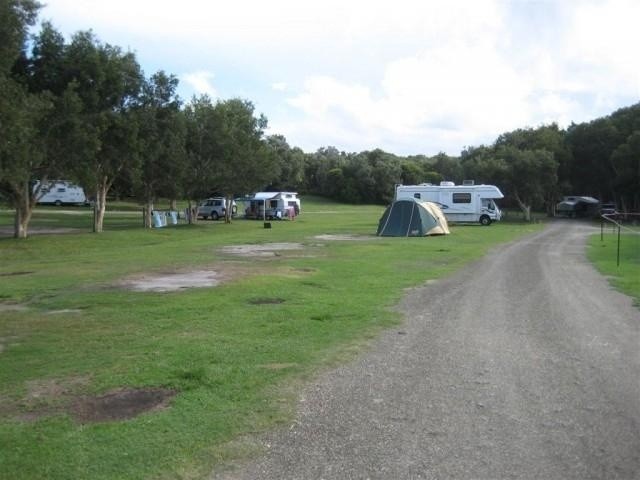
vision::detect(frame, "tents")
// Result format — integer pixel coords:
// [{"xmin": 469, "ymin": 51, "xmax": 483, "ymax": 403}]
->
[{"xmin": 375, "ymin": 197, "xmax": 451, "ymax": 237}]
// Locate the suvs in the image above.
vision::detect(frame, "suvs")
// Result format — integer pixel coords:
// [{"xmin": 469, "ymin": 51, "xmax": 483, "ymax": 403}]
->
[{"xmin": 193, "ymin": 196, "xmax": 238, "ymax": 221}]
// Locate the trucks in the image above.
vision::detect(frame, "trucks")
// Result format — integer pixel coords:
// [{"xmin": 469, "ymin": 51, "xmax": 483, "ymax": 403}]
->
[
  {"xmin": 396, "ymin": 180, "xmax": 505, "ymax": 226},
  {"xmin": 31, "ymin": 179, "xmax": 94, "ymax": 207}
]
